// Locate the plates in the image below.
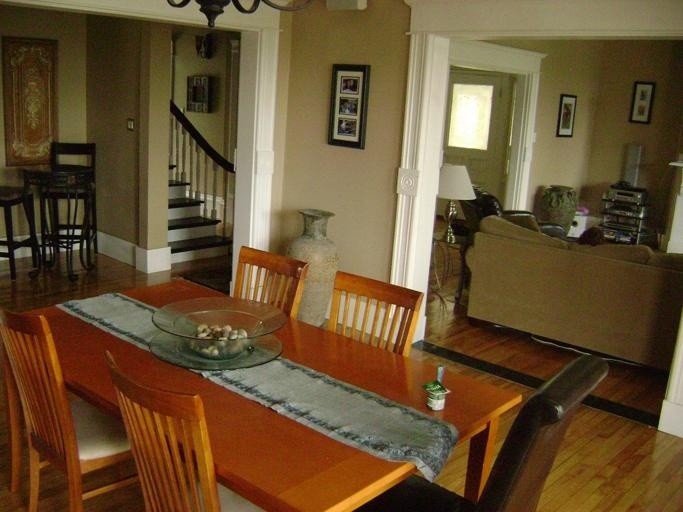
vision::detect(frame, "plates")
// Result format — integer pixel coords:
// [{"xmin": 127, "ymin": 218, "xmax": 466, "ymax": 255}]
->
[{"xmin": 150, "ymin": 331, "xmax": 285, "ymax": 371}]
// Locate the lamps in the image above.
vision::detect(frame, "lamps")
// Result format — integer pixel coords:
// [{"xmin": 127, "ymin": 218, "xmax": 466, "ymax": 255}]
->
[
  {"xmin": 193, "ymin": 31, "xmax": 215, "ymax": 61},
  {"xmin": 161, "ymin": 0, "xmax": 316, "ymax": 28},
  {"xmin": 435, "ymin": 161, "xmax": 476, "ymax": 244}
]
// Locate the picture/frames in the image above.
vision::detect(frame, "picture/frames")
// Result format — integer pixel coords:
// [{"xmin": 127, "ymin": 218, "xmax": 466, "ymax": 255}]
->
[
  {"xmin": 555, "ymin": 93, "xmax": 577, "ymax": 137},
  {"xmin": 326, "ymin": 63, "xmax": 371, "ymax": 151},
  {"xmin": 628, "ymin": 80, "xmax": 656, "ymax": 124}
]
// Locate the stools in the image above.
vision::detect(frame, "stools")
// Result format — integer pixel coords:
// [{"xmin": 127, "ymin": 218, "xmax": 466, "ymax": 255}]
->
[{"xmin": 0, "ymin": 184, "xmax": 42, "ymax": 280}]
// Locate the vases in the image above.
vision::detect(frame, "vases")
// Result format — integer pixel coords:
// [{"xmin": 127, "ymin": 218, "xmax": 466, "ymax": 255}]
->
[{"xmin": 282, "ymin": 207, "xmax": 340, "ymax": 327}]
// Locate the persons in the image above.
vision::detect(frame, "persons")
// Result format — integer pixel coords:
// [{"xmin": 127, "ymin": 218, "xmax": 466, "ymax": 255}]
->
[
  {"xmin": 579, "ymin": 226, "xmax": 605, "ymax": 246},
  {"xmin": 340, "ymin": 80, "xmax": 357, "ymax": 134}
]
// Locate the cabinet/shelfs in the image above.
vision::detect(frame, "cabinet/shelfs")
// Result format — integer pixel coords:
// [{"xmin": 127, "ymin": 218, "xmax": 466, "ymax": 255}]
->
[{"xmin": 598, "ymin": 197, "xmax": 649, "ymax": 246}]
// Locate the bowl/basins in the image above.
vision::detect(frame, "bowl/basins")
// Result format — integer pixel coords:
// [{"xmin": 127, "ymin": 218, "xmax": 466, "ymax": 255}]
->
[{"xmin": 151, "ymin": 296, "xmax": 288, "ymax": 358}]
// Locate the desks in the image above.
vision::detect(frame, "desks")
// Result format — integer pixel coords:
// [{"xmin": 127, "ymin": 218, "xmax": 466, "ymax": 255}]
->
[
  {"xmin": 1, "ymin": 276, "xmax": 524, "ymax": 511},
  {"xmin": 19, "ymin": 165, "xmax": 97, "ymax": 281}
]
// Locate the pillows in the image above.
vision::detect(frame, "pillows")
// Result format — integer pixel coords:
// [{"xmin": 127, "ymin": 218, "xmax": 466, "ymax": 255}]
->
[{"xmin": 502, "ymin": 213, "xmax": 541, "ymax": 237}]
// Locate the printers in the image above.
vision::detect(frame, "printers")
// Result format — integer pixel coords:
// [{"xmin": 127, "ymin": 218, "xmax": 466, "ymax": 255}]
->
[{"xmin": 608, "ymin": 188, "xmax": 649, "ymax": 204}]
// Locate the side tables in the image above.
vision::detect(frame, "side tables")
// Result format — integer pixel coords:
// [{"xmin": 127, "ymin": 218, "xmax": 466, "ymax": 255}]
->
[{"xmin": 427, "ymin": 230, "xmax": 464, "ymax": 312}]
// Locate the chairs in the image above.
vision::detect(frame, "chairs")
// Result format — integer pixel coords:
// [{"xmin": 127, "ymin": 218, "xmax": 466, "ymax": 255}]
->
[
  {"xmin": 235, "ymin": 245, "xmax": 310, "ymax": 321},
  {"xmin": 347, "ymin": 351, "xmax": 609, "ymax": 510},
  {"xmin": 0, "ymin": 309, "xmax": 140, "ymax": 510},
  {"xmin": 453, "ymin": 187, "xmax": 566, "ymax": 307},
  {"xmin": 328, "ymin": 270, "xmax": 424, "ymax": 357},
  {"xmin": 104, "ymin": 348, "xmax": 262, "ymax": 511},
  {"xmin": 36, "ymin": 141, "xmax": 97, "ymax": 255}
]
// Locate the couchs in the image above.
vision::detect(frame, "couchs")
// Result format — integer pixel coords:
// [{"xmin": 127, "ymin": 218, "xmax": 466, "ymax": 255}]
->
[{"xmin": 463, "ymin": 212, "xmax": 683, "ymax": 375}]
